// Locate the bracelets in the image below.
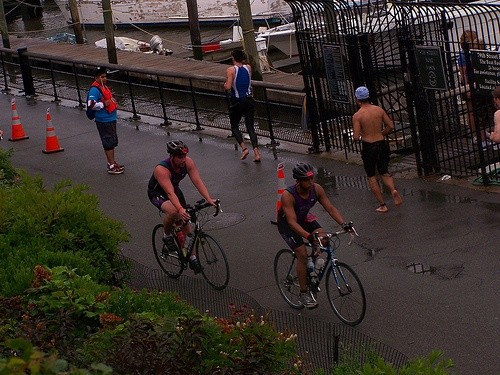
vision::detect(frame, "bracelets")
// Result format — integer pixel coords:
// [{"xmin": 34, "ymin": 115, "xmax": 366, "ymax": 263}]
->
[
  {"xmin": 224, "ymin": 48, "xmax": 261, "ymax": 162},
  {"xmin": 176, "ymin": 205, "xmax": 182, "ymax": 213}
]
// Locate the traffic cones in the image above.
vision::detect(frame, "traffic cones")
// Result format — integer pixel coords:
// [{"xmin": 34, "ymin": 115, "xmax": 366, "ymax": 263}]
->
[
  {"xmin": 7, "ymin": 97, "xmax": 30, "ymax": 141},
  {"xmin": 41, "ymin": 110, "xmax": 65, "ymax": 154},
  {"xmin": 269, "ymin": 162, "xmax": 285, "ymax": 226}
]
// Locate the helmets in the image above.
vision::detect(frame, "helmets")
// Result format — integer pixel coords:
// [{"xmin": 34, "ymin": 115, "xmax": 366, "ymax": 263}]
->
[
  {"xmin": 292, "ymin": 162, "xmax": 316, "ymax": 178},
  {"xmin": 166, "ymin": 139, "xmax": 189, "ymax": 154}
]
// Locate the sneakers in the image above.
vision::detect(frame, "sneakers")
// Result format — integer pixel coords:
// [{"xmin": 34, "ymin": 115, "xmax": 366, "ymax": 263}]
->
[
  {"xmin": 106, "ymin": 162, "xmax": 125, "ymax": 169},
  {"xmin": 298, "ymin": 292, "xmax": 318, "ymax": 308},
  {"xmin": 189, "ymin": 258, "xmax": 202, "ymax": 274},
  {"xmin": 108, "ymin": 164, "xmax": 123, "ymax": 174},
  {"xmin": 162, "ymin": 233, "xmax": 178, "ymax": 251}
]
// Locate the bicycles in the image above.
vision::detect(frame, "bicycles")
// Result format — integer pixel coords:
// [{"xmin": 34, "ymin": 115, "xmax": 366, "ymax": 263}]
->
[
  {"xmin": 151, "ymin": 199, "xmax": 231, "ymax": 291},
  {"xmin": 274, "ymin": 220, "xmax": 368, "ymax": 327}
]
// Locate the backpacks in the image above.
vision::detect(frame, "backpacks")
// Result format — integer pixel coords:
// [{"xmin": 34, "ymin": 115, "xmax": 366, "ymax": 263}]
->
[{"xmin": 85, "ymin": 86, "xmax": 104, "ymax": 120}]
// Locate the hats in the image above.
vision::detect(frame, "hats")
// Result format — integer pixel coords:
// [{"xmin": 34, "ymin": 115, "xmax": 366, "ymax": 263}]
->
[
  {"xmin": 95, "ymin": 66, "xmax": 107, "ymax": 75},
  {"xmin": 356, "ymin": 87, "xmax": 369, "ymax": 99}
]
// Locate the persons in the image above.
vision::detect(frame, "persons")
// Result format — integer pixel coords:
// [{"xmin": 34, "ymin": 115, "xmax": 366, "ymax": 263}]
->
[
  {"xmin": 457, "ymin": 30, "xmax": 500, "ymax": 149},
  {"xmin": 277, "ymin": 162, "xmax": 353, "ymax": 309},
  {"xmin": 147, "ymin": 140, "xmax": 221, "ymax": 274},
  {"xmin": 86, "ymin": 68, "xmax": 125, "ymax": 174},
  {"xmin": 352, "ymin": 86, "xmax": 402, "ymax": 212}
]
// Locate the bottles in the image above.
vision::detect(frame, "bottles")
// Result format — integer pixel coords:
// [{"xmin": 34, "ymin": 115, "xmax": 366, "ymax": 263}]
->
[
  {"xmin": 315, "ymin": 259, "xmax": 324, "ymax": 274},
  {"xmin": 306, "ymin": 256, "xmax": 315, "ymax": 276},
  {"xmin": 177, "ymin": 229, "xmax": 185, "ymax": 248},
  {"xmin": 185, "ymin": 232, "xmax": 192, "ymax": 249}
]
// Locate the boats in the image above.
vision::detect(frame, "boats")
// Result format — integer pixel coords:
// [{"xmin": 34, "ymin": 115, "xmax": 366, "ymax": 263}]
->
[
  {"xmin": 176, "ymin": 17, "xmax": 328, "ymax": 65},
  {"xmin": 48, "ymin": 32, "xmax": 87, "ymax": 45},
  {"xmin": 95, "ymin": 33, "xmax": 174, "ymax": 55}
]
